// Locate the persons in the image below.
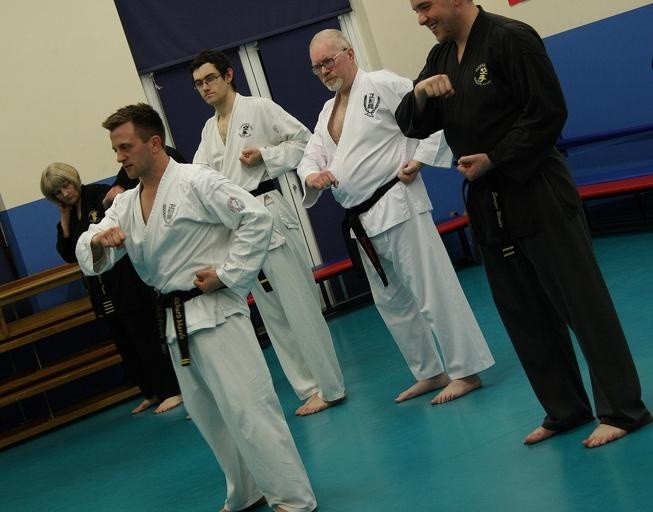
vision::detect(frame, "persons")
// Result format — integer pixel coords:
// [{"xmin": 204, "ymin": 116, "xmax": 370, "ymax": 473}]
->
[
  {"xmin": 40, "ymin": 162, "xmax": 181, "ymax": 411},
  {"xmin": 187, "ymin": 49, "xmax": 346, "ymax": 415},
  {"xmin": 102, "ymin": 145, "xmax": 191, "ymax": 421},
  {"xmin": 75, "ymin": 104, "xmax": 319, "ymax": 512},
  {"xmin": 395, "ymin": 1, "xmax": 653, "ymax": 448},
  {"xmin": 297, "ymin": 28, "xmax": 496, "ymax": 405}
]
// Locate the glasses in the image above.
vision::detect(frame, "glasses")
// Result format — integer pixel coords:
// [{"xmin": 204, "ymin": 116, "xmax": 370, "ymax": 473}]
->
[
  {"xmin": 193, "ymin": 74, "xmax": 221, "ymax": 90},
  {"xmin": 311, "ymin": 48, "xmax": 346, "ymax": 76}
]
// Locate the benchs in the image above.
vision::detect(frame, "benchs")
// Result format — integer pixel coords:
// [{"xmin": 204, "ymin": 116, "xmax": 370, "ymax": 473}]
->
[
  {"xmin": 562, "ymin": 131, "xmax": 653, "ymax": 235},
  {"xmin": 246, "ymin": 213, "xmax": 469, "ymax": 344}
]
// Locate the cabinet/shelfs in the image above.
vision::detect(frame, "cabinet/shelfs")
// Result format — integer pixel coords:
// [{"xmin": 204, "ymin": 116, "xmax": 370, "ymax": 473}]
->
[{"xmin": 0, "ymin": 260, "xmax": 141, "ymax": 453}]
